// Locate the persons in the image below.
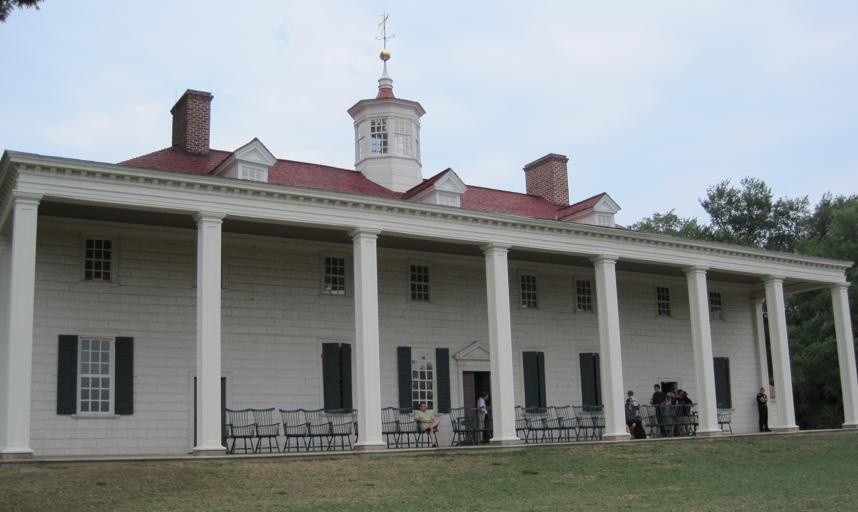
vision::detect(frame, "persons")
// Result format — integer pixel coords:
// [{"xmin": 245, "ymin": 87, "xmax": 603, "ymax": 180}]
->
[
  {"xmin": 412, "ymin": 403, "xmax": 440, "ymax": 448},
  {"xmin": 477, "ymin": 392, "xmax": 489, "ymax": 439},
  {"xmin": 624, "ymin": 384, "xmax": 693, "ymax": 440},
  {"xmin": 757, "ymin": 386, "xmax": 772, "ymax": 433}
]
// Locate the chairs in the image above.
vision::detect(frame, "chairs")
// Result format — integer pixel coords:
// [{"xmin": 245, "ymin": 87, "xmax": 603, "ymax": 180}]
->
[
  {"xmin": 222, "ymin": 407, "xmax": 440, "ymax": 455},
  {"xmin": 625, "ymin": 401, "xmax": 734, "ymax": 440},
  {"xmin": 446, "ymin": 406, "xmax": 605, "ymax": 446}
]
